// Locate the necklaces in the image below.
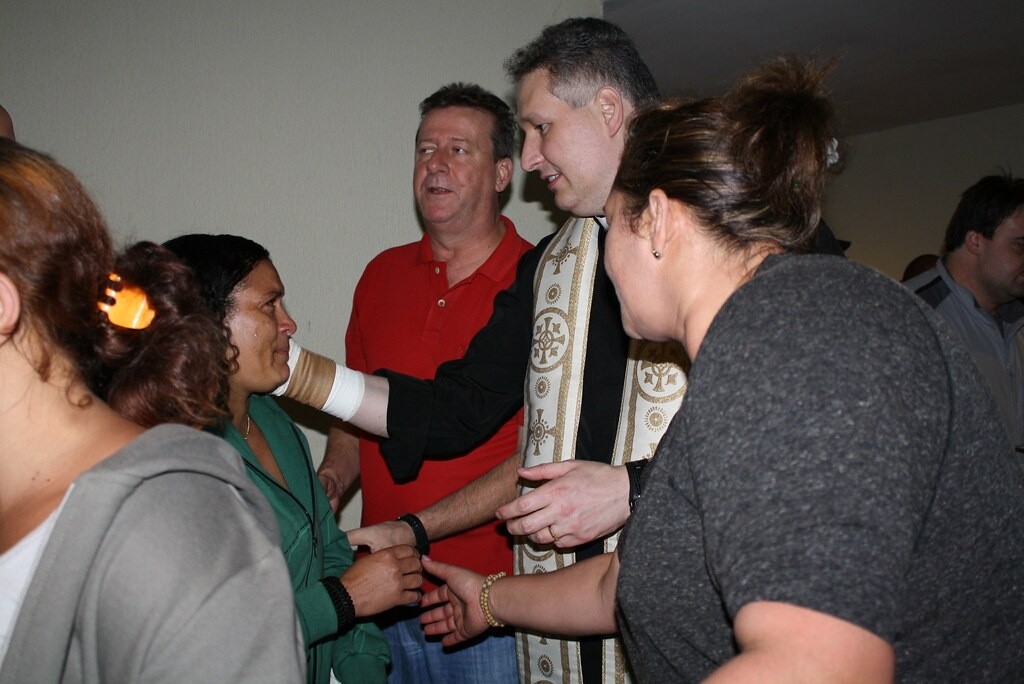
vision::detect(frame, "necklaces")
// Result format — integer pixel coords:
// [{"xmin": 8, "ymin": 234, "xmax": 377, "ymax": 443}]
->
[{"xmin": 244, "ymin": 412, "xmax": 252, "ymax": 441}]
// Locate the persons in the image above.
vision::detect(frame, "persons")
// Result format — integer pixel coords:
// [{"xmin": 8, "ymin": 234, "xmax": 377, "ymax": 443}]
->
[
  {"xmin": 317, "ymin": 83, "xmax": 535, "ymax": 684},
  {"xmin": 0, "ymin": 140, "xmax": 309, "ymax": 684},
  {"xmin": 273, "ymin": 15, "xmax": 690, "ymax": 684},
  {"xmin": 123, "ymin": 229, "xmax": 422, "ymax": 683},
  {"xmin": 783, "ymin": 164, "xmax": 1024, "ymax": 495},
  {"xmin": 418, "ymin": 43, "xmax": 1024, "ymax": 684}
]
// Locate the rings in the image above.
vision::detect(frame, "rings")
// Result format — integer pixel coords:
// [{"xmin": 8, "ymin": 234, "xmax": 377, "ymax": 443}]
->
[{"xmin": 548, "ymin": 526, "xmax": 559, "ymax": 542}]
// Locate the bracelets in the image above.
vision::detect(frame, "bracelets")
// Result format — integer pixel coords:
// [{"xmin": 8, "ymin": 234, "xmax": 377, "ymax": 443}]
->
[
  {"xmin": 624, "ymin": 458, "xmax": 653, "ymax": 516},
  {"xmin": 480, "ymin": 572, "xmax": 507, "ymax": 628},
  {"xmin": 395, "ymin": 512, "xmax": 430, "ymax": 558}
]
